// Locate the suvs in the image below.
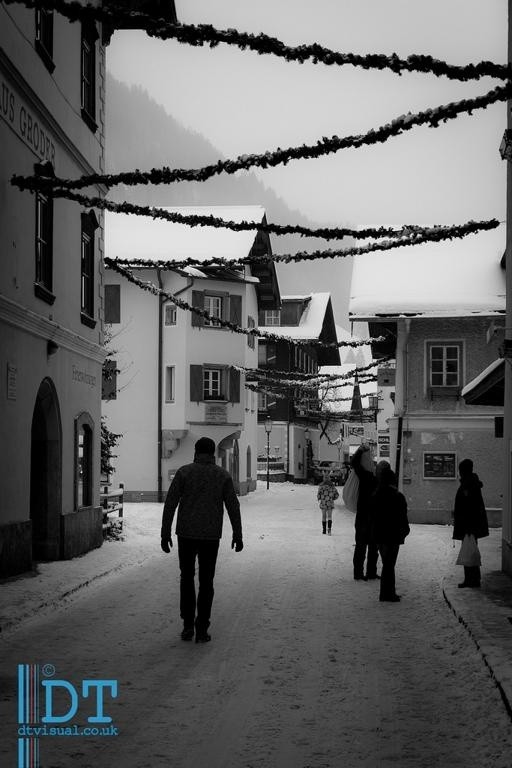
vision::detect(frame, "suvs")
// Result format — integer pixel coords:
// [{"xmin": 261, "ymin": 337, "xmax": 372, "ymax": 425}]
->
[{"xmin": 309, "ymin": 460, "xmax": 348, "ymax": 486}]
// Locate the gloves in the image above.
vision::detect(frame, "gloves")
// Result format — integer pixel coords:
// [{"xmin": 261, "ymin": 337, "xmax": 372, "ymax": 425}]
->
[
  {"xmin": 231, "ymin": 539, "xmax": 243, "ymax": 552},
  {"xmin": 161, "ymin": 536, "xmax": 172, "ymax": 552}
]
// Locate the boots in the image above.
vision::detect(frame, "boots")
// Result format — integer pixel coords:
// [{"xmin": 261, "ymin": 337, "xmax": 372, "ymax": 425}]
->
[{"xmin": 322, "ymin": 520, "xmax": 332, "ymax": 536}]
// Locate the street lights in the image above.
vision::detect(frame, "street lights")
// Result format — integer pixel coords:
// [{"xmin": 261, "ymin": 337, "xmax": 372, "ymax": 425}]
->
[
  {"xmin": 304, "ymin": 428, "xmax": 311, "ymax": 484},
  {"xmin": 263, "ymin": 415, "xmax": 273, "ymax": 490}
]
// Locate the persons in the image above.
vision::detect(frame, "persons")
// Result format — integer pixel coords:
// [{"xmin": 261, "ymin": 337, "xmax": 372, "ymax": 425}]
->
[
  {"xmin": 452, "ymin": 459, "xmax": 489, "ymax": 588},
  {"xmin": 348, "ymin": 442, "xmax": 382, "ymax": 581},
  {"xmin": 375, "ymin": 470, "xmax": 410, "ymax": 601},
  {"xmin": 317, "ymin": 474, "xmax": 339, "ymax": 536},
  {"xmin": 160, "ymin": 437, "xmax": 243, "ymax": 644}
]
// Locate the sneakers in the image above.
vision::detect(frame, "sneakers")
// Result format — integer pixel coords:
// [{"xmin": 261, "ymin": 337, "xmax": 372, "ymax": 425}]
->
[
  {"xmin": 380, "ymin": 593, "xmax": 401, "ymax": 603},
  {"xmin": 181, "ymin": 631, "xmax": 211, "ymax": 643},
  {"xmin": 355, "ymin": 573, "xmax": 381, "ymax": 581}
]
